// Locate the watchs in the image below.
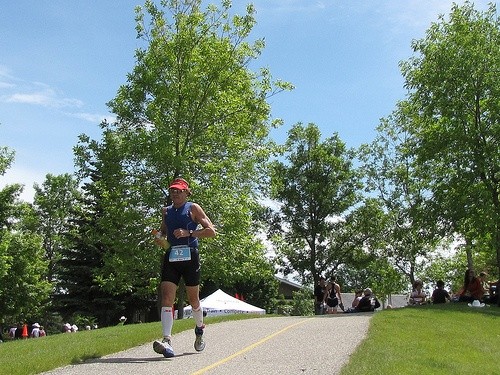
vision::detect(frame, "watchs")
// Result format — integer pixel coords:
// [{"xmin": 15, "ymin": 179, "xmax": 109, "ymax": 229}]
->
[{"xmin": 189, "ymin": 229, "xmax": 193, "ymax": 238}]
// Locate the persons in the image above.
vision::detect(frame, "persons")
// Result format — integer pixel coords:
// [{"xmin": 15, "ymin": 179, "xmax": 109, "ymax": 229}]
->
[
  {"xmin": 63, "ymin": 323, "xmax": 78, "ymax": 333},
  {"xmin": 450, "ymin": 269, "xmax": 500, "ymax": 302},
  {"xmin": 408, "ymin": 279, "xmax": 427, "ymax": 307},
  {"xmin": 431, "ymin": 280, "xmax": 452, "ymax": 304},
  {"xmin": 349, "ymin": 288, "xmax": 380, "ymax": 312},
  {"xmin": 153, "ymin": 178, "xmax": 217, "ymax": 358},
  {"xmin": 30, "ymin": 322, "xmax": 46, "ymax": 338},
  {"xmin": 314, "ymin": 276, "xmax": 343, "ymax": 315},
  {"xmin": 14, "ymin": 319, "xmax": 28, "ymax": 341}
]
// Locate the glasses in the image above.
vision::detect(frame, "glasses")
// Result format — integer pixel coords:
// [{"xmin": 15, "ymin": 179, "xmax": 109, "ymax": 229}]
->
[{"xmin": 172, "ymin": 189, "xmax": 186, "ymax": 194}]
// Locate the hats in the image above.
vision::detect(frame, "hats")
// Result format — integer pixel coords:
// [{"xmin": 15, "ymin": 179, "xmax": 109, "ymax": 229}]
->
[
  {"xmin": 468, "ymin": 300, "xmax": 485, "ymax": 307},
  {"xmin": 121, "ymin": 316, "xmax": 125, "ymax": 320},
  {"xmin": 63, "ymin": 323, "xmax": 71, "ymax": 329},
  {"xmin": 365, "ymin": 288, "xmax": 372, "ymax": 295},
  {"xmin": 170, "ymin": 179, "xmax": 189, "ymax": 190},
  {"xmin": 32, "ymin": 323, "xmax": 40, "ymax": 327},
  {"xmin": 71, "ymin": 325, "xmax": 78, "ymax": 330}
]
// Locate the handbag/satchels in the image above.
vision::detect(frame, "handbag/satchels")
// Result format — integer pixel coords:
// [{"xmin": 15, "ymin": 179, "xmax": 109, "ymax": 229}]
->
[{"xmin": 326, "ymin": 297, "xmax": 339, "ymax": 308}]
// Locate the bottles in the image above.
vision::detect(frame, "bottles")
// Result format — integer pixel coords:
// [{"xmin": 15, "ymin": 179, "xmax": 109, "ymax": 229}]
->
[{"xmin": 152, "ymin": 229, "xmax": 171, "ymax": 250}]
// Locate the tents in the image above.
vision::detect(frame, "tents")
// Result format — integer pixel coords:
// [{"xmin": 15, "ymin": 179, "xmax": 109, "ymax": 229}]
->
[{"xmin": 174, "ymin": 288, "xmax": 266, "ymax": 319}]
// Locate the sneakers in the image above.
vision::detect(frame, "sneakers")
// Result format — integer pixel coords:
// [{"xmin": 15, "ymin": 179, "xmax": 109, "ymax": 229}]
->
[
  {"xmin": 153, "ymin": 337, "xmax": 174, "ymax": 358},
  {"xmin": 194, "ymin": 334, "xmax": 205, "ymax": 351}
]
